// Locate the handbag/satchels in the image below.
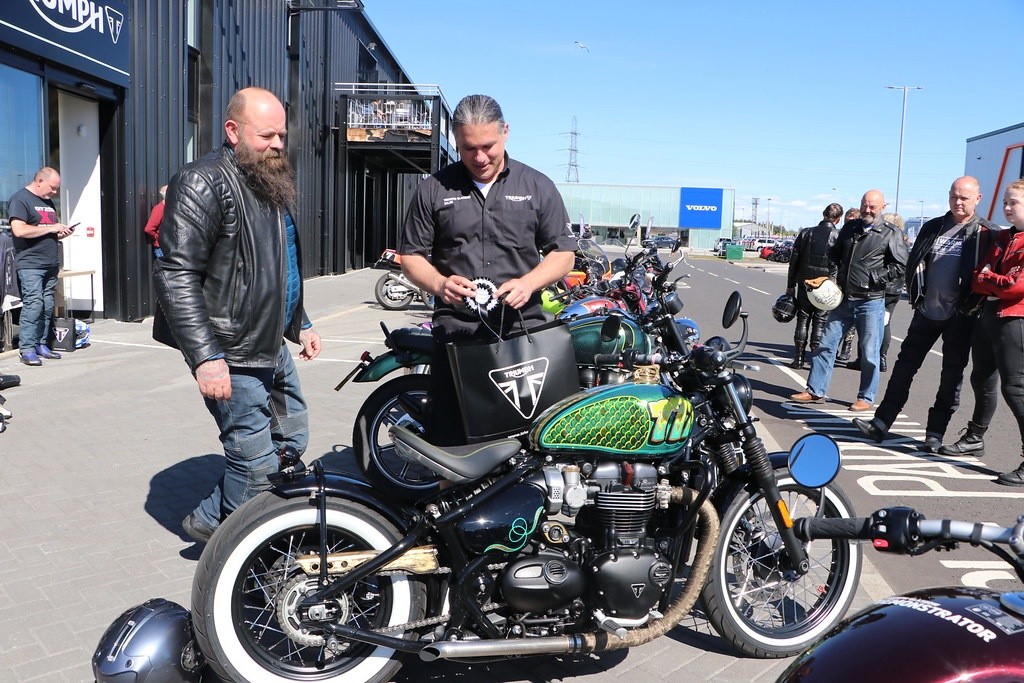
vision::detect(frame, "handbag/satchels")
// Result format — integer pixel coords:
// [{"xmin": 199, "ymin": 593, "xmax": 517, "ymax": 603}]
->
[{"xmin": 446, "ymin": 299, "xmax": 580, "ymax": 445}]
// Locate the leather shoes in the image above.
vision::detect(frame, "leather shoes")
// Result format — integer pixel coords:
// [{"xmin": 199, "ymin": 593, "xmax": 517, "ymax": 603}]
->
[
  {"xmin": 924, "ymin": 435, "xmax": 941, "ymax": 453},
  {"xmin": 850, "ymin": 400, "xmax": 873, "ymax": 410},
  {"xmin": 852, "ymin": 418, "xmax": 886, "ymax": 444},
  {"xmin": 790, "ymin": 390, "xmax": 825, "ymax": 403}
]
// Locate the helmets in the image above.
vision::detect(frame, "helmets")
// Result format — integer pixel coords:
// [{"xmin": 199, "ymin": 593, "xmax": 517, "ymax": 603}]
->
[
  {"xmin": 806, "ymin": 277, "xmax": 844, "ymax": 311},
  {"xmin": 772, "ymin": 295, "xmax": 798, "ymax": 323}
]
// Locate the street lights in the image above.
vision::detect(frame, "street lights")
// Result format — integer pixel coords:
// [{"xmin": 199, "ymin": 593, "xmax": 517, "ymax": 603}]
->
[
  {"xmin": 767, "ymin": 198, "xmax": 771, "ymax": 238},
  {"xmin": 886, "ymin": 86, "xmax": 922, "ymax": 215}
]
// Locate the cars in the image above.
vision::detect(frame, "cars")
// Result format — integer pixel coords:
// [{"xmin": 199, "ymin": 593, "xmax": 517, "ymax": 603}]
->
[
  {"xmin": 640, "ymin": 235, "xmax": 678, "ymax": 249},
  {"xmin": 742, "ymin": 237, "xmax": 777, "ymax": 251},
  {"xmin": 714, "ymin": 237, "xmax": 731, "ymax": 251}
]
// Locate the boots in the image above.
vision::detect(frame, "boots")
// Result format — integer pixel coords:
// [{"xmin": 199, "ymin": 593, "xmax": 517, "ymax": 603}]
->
[
  {"xmin": 790, "ymin": 340, "xmax": 808, "ymax": 369},
  {"xmin": 998, "ymin": 445, "xmax": 1024, "ymax": 487},
  {"xmin": 941, "ymin": 421, "xmax": 989, "ymax": 456},
  {"xmin": 837, "ymin": 326, "xmax": 855, "ymax": 360},
  {"xmin": 808, "ymin": 343, "xmax": 818, "ymax": 365}
]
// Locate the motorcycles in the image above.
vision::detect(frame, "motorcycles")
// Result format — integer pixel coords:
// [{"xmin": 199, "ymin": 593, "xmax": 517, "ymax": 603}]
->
[
  {"xmin": 190, "ymin": 207, "xmax": 864, "ymax": 683},
  {"xmin": 767, "ymin": 243, "xmax": 792, "ymax": 263},
  {"xmin": 777, "ymin": 507, "xmax": 1024, "ymax": 683}
]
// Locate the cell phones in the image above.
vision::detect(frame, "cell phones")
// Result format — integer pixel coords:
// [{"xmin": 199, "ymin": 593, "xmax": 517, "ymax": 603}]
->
[{"xmin": 68, "ymin": 222, "xmax": 81, "ymax": 230}]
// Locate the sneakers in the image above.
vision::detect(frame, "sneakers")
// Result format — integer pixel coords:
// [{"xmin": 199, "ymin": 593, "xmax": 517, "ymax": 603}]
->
[
  {"xmin": 35, "ymin": 343, "xmax": 61, "ymax": 359},
  {"xmin": 19, "ymin": 348, "xmax": 42, "ymax": 366},
  {"xmin": 183, "ymin": 513, "xmax": 215, "ymax": 542}
]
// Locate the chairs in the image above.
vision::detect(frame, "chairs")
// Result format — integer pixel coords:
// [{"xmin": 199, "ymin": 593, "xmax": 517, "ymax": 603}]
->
[{"xmin": 360, "ymin": 105, "xmax": 386, "ymax": 128}]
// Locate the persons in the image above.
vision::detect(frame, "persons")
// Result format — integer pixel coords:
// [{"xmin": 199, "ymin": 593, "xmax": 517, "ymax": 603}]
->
[
  {"xmin": 150, "ymin": 86, "xmax": 323, "ymax": 545},
  {"xmin": 7, "ymin": 165, "xmax": 76, "ymax": 367},
  {"xmin": 398, "ymin": 271, "xmax": 399, "ymax": 273},
  {"xmin": 399, "ymin": 93, "xmax": 579, "ymax": 489},
  {"xmin": 850, "ymin": 175, "xmax": 1004, "ymax": 453},
  {"xmin": 144, "ymin": 184, "xmax": 171, "ymax": 261},
  {"xmin": 785, "ymin": 189, "xmax": 910, "ymax": 413},
  {"xmin": 938, "ymin": 178, "xmax": 1024, "ymax": 486}
]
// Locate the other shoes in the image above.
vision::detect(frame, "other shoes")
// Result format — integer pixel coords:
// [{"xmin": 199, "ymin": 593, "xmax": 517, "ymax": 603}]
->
[
  {"xmin": 880, "ymin": 354, "xmax": 887, "ymax": 372},
  {"xmin": 847, "ymin": 359, "xmax": 864, "ymax": 370}
]
